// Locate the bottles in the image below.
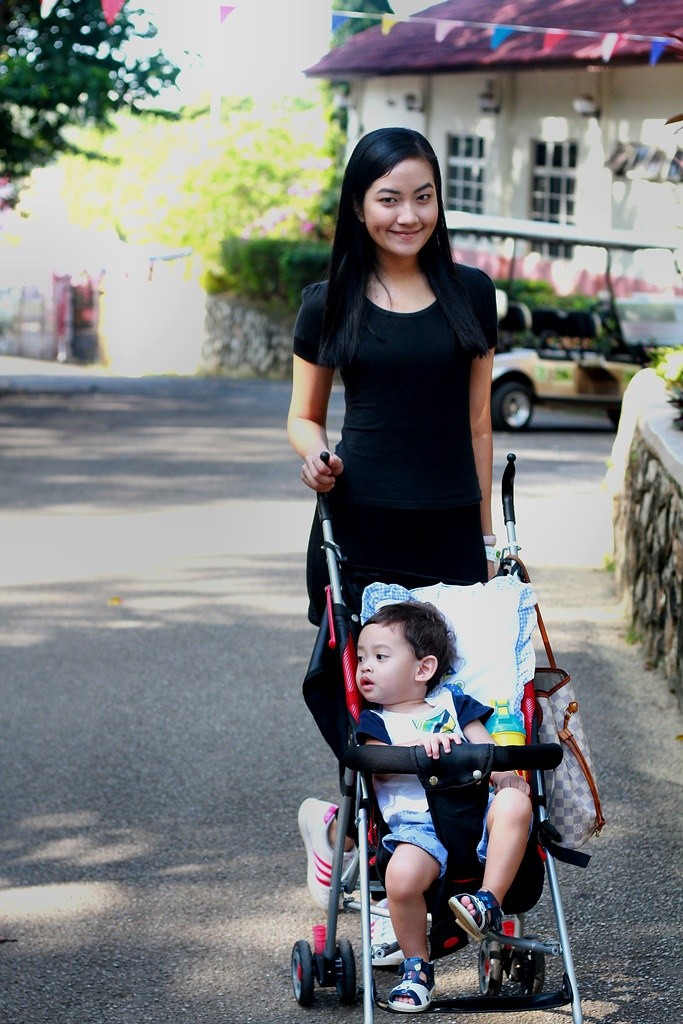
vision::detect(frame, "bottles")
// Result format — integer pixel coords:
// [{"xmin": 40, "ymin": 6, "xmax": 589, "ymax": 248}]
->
[{"xmin": 485, "ymin": 699, "xmax": 525, "ymax": 782}]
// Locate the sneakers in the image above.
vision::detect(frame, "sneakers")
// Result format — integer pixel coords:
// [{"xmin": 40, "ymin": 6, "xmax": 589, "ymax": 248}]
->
[
  {"xmin": 370, "ymin": 899, "xmax": 403, "ymax": 967},
  {"xmin": 297, "ymin": 798, "xmax": 359, "ymax": 914}
]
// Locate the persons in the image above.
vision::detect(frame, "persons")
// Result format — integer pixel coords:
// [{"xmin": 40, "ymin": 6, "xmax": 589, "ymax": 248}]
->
[
  {"xmin": 354, "ymin": 601, "xmax": 533, "ymax": 1012},
  {"xmin": 288, "ymin": 129, "xmax": 496, "ymax": 965}
]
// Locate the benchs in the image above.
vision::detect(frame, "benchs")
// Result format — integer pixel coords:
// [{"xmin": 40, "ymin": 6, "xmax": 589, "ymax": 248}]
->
[{"xmin": 498, "ymin": 300, "xmax": 607, "ymax": 361}]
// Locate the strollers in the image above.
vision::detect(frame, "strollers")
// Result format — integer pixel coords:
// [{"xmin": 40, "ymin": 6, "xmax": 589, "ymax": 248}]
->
[{"xmin": 317, "ymin": 448, "xmax": 585, "ymax": 1023}]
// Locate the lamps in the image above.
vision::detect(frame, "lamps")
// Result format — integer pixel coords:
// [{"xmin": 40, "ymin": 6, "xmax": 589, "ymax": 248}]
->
[
  {"xmin": 479, "ymin": 90, "xmax": 501, "ymax": 115},
  {"xmin": 403, "ymin": 92, "xmax": 426, "ymax": 113},
  {"xmin": 572, "ymin": 91, "xmax": 602, "ymax": 118}
]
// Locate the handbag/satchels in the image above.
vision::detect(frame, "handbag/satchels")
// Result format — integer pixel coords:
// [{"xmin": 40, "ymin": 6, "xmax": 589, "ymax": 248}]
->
[{"xmin": 499, "ymin": 553, "xmax": 604, "ymax": 850}]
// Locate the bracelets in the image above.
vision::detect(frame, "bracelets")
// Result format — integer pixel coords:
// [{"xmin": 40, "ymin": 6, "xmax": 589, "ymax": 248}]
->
[{"xmin": 481, "ymin": 534, "xmax": 500, "ymax": 565}]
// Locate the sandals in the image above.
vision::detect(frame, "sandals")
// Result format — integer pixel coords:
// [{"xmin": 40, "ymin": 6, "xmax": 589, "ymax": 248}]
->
[
  {"xmin": 449, "ymin": 887, "xmax": 503, "ymax": 940},
  {"xmin": 387, "ymin": 954, "xmax": 436, "ymax": 1013}
]
